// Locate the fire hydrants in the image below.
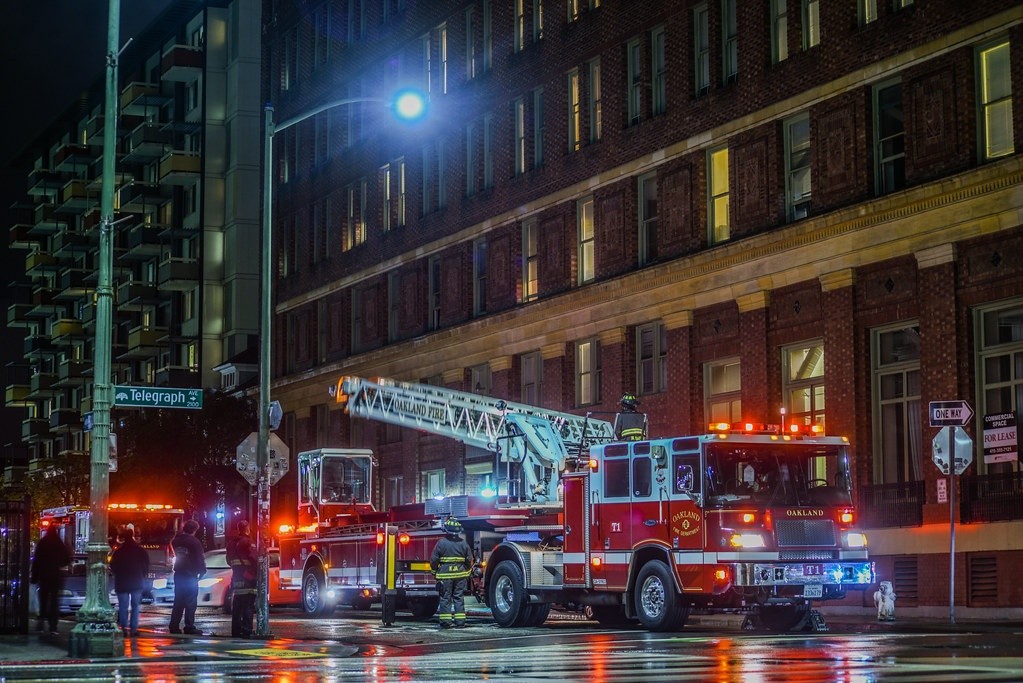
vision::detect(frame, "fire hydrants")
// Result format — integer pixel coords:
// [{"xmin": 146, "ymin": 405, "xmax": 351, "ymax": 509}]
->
[{"xmin": 874, "ymin": 581, "xmax": 897, "ymax": 621}]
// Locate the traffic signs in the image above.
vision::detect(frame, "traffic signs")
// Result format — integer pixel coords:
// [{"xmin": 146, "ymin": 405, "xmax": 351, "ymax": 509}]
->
[{"xmin": 929, "ymin": 400, "xmax": 974, "ymax": 427}]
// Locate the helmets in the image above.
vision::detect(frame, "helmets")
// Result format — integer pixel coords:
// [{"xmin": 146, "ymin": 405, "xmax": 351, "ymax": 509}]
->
[
  {"xmin": 443, "ymin": 517, "xmax": 465, "ymax": 536},
  {"xmin": 620, "ymin": 392, "xmax": 639, "ymax": 405}
]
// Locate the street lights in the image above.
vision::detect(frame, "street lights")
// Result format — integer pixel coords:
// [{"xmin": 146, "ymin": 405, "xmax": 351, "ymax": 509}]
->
[{"xmin": 257, "ymin": 95, "xmax": 421, "ymax": 641}]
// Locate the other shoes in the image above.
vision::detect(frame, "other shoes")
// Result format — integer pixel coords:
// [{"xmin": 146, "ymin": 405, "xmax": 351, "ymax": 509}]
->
[
  {"xmin": 34, "ymin": 626, "xmax": 44, "ymax": 633},
  {"xmin": 169, "ymin": 626, "xmax": 182, "ymax": 634},
  {"xmin": 184, "ymin": 625, "xmax": 203, "ymax": 636},
  {"xmin": 131, "ymin": 631, "xmax": 139, "ymax": 637},
  {"xmin": 49, "ymin": 627, "xmax": 59, "ymax": 634}
]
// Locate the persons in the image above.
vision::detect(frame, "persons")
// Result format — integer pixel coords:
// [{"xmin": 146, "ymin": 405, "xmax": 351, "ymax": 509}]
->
[
  {"xmin": 430, "ymin": 518, "xmax": 474, "ymax": 628},
  {"xmin": 169, "ymin": 521, "xmax": 207, "ymax": 635},
  {"xmin": 615, "ymin": 395, "xmax": 648, "ymax": 441},
  {"xmin": 227, "ymin": 520, "xmax": 258, "ymax": 638},
  {"xmin": 110, "ymin": 529, "xmax": 149, "ymax": 634},
  {"xmin": 31, "ymin": 526, "xmax": 69, "ymax": 635}
]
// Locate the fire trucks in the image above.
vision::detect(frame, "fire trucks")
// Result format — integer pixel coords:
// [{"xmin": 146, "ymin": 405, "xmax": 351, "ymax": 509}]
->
[
  {"xmin": 278, "ymin": 374, "xmax": 876, "ymax": 633},
  {"xmin": 29, "ymin": 503, "xmax": 185, "ymax": 616}
]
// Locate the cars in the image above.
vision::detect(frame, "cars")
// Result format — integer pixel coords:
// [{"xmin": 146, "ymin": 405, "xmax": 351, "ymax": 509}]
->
[{"xmin": 151, "ymin": 547, "xmax": 303, "ymax": 615}]
